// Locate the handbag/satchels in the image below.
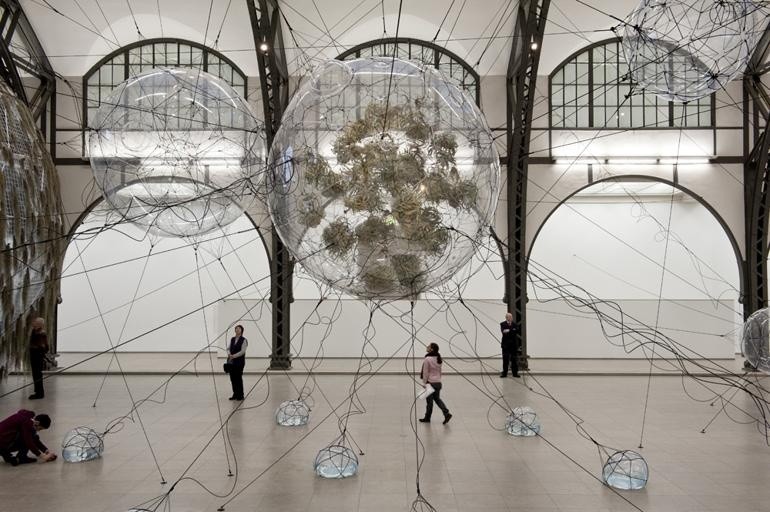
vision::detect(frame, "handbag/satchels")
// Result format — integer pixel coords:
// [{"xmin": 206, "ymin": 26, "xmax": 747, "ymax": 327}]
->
[
  {"xmin": 224, "ymin": 363, "xmax": 230, "ymax": 373},
  {"xmin": 418, "ymin": 384, "xmax": 435, "ymax": 400}
]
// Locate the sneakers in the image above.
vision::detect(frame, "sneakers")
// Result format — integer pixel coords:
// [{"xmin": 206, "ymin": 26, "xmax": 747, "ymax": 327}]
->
[
  {"xmin": 513, "ymin": 374, "xmax": 520, "ymax": 378},
  {"xmin": 29, "ymin": 392, "xmax": 44, "ymax": 400},
  {"xmin": 229, "ymin": 395, "xmax": 244, "ymax": 401},
  {"xmin": 500, "ymin": 375, "xmax": 507, "ymax": 378},
  {"xmin": 443, "ymin": 413, "xmax": 452, "ymax": 425},
  {"xmin": 419, "ymin": 419, "xmax": 430, "ymax": 422},
  {"xmin": 2, "ymin": 453, "xmax": 37, "ymax": 466}
]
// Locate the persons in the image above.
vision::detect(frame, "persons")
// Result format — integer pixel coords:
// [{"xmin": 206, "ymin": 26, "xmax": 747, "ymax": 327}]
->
[
  {"xmin": 225, "ymin": 324, "xmax": 249, "ymax": 402},
  {"xmin": 1, "ymin": 408, "xmax": 58, "ymax": 467},
  {"xmin": 420, "ymin": 342, "xmax": 453, "ymax": 424},
  {"xmin": 29, "ymin": 317, "xmax": 50, "ymax": 398},
  {"xmin": 498, "ymin": 312, "xmax": 522, "ymax": 378}
]
